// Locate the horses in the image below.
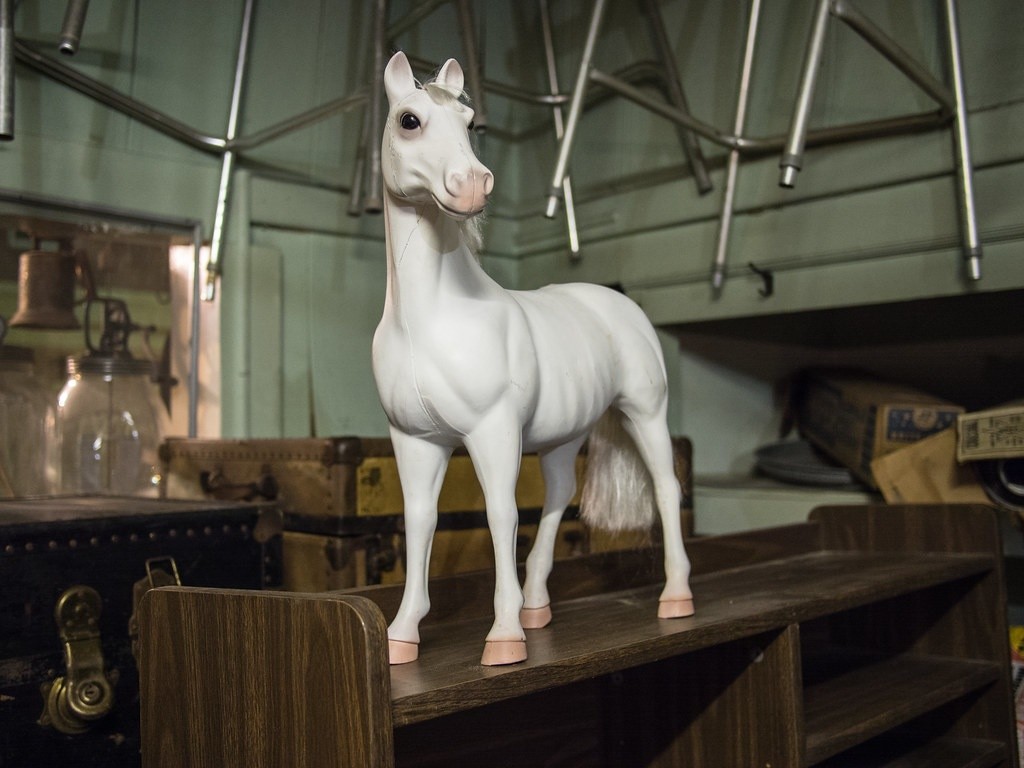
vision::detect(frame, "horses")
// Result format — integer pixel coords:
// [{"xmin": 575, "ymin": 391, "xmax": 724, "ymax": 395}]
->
[{"xmin": 370, "ymin": 48, "xmax": 695, "ymax": 666}]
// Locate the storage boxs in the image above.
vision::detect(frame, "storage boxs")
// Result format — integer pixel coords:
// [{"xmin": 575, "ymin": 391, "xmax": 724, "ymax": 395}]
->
[
  {"xmin": 0, "ymin": 493, "xmax": 285, "ymax": 768},
  {"xmin": 807, "ymin": 381, "xmax": 963, "ymax": 491},
  {"xmin": 957, "ymin": 399, "xmax": 1024, "ymax": 463},
  {"xmin": 160, "ymin": 435, "xmax": 696, "ymax": 590}
]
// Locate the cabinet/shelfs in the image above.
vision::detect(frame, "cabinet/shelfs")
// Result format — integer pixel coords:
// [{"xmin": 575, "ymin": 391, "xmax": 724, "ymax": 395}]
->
[{"xmin": 139, "ymin": 501, "xmax": 1020, "ymax": 768}]
